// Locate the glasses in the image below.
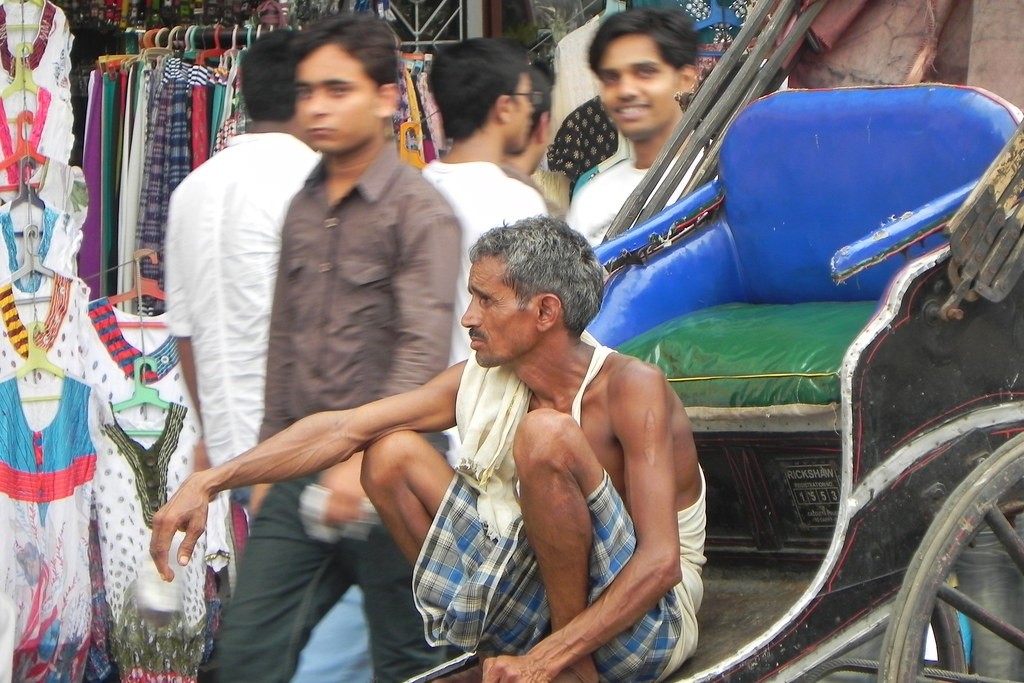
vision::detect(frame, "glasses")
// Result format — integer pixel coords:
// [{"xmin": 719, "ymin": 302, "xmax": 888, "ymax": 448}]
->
[{"xmin": 505, "ymin": 91, "xmax": 546, "ymax": 105}]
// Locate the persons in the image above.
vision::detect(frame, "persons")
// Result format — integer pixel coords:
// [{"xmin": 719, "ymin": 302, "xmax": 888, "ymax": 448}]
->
[
  {"xmin": 500, "ymin": 66, "xmax": 563, "ymax": 221},
  {"xmin": 150, "ymin": 218, "xmax": 707, "ymax": 683},
  {"xmin": 417, "ymin": 39, "xmax": 548, "ymax": 436},
  {"xmin": 567, "ymin": 6, "xmax": 721, "ymax": 246},
  {"xmin": 165, "ymin": 30, "xmax": 374, "ymax": 683},
  {"xmin": 203, "ymin": 13, "xmax": 460, "ymax": 683}
]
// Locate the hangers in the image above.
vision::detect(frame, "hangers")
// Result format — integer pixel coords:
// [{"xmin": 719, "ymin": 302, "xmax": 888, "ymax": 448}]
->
[{"xmin": 79, "ymin": 21, "xmax": 449, "ymax": 65}]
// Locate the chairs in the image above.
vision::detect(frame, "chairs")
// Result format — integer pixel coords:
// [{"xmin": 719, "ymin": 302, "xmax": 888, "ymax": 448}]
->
[{"xmin": 584, "ymin": 79, "xmax": 1023, "ymax": 570}]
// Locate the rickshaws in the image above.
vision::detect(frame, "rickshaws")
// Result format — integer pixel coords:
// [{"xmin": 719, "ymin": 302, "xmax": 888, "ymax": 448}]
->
[{"xmin": 404, "ymin": 79, "xmax": 1024, "ymax": 683}]
